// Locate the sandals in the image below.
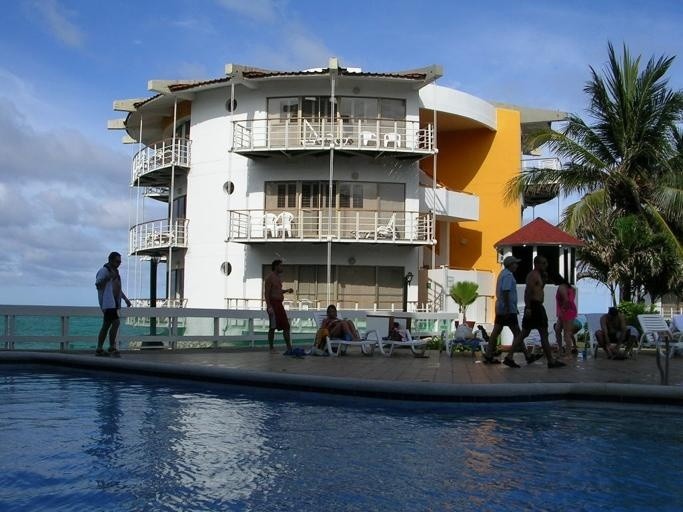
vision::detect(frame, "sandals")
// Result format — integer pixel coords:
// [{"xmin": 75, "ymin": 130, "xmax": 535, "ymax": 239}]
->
[
  {"xmin": 547, "ymin": 359, "xmax": 568, "ymax": 369},
  {"xmin": 502, "ymin": 356, "xmax": 520, "ymax": 369},
  {"xmin": 525, "ymin": 353, "xmax": 543, "ymax": 365},
  {"xmin": 481, "ymin": 353, "xmax": 501, "ymax": 366}
]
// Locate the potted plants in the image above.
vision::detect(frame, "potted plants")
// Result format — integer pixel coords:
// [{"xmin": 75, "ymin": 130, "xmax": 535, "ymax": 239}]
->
[{"xmin": 449, "ymin": 281, "xmax": 479, "ymax": 331}]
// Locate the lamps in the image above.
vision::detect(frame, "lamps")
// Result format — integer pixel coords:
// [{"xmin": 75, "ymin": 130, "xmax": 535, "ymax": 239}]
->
[{"xmin": 403, "ymin": 271, "xmax": 414, "ymax": 285}]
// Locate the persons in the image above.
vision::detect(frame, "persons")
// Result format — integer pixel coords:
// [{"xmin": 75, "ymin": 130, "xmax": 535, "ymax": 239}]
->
[
  {"xmin": 552, "ymin": 319, "xmax": 582, "ymax": 355},
  {"xmin": 594, "ymin": 307, "xmax": 638, "ymax": 361},
  {"xmin": 548, "ymin": 268, "xmax": 578, "ymax": 361},
  {"xmin": 320, "ymin": 304, "xmax": 363, "ymax": 342},
  {"xmin": 263, "ymin": 259, "xmax": 295, "ymax": 354},
  {"xmin": 486, "ymin": 254, "xmax": 544, "ymax": 365},
  {"xmin": 94, "ymin": 250, "xmax": 131, "ymax": 354},
  {"xmin": 502, "ymin": 255, "xmax": 568, "ymax": 370}
]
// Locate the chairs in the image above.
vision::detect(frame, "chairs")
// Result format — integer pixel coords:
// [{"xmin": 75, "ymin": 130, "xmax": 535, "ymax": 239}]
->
[
  {"xmin": 299, "ymin": 118, "xmax": 353, "ymax": 147},
  {"xmin": 353, "ymin": 212, "xmax": 400, "ymax": 240},
  {"xmin": 313, "ymin": 310, "xmax": 376, "ymax": 357},
  {"xmin": 358, "ymin": 131, "xmax": 377, "ymax": 146},
  {"xmin": 586, "ymin": 310, "xmax": 634, "ymax": 359},
  {"xmin": 270, "ymin": 212, "xmax": 294, "ymax": 238},
  {"xmin": 637, "ymin": 314, "xmax": 682, "ymax": 358},
  {"xmin": 132, "ymin": 146, "xmax": 179, "ymax": 178},
  {"xmin": 270, "ymin": 296, "xmax": 316, "ymax": 328},
  {"xmin": 383, "ymin": 133, "xmax": 401, "ymax": 147},
  {"xmin": 134, "ymin": 298, "xmax": 180, "ymax": 325},
  {"xmin": 441, "ymin": 329, "xmax": 488, "ymax": 357},
  {"xmin": 516, "ymin": 303, "xmax": 559, "ymax": 355},
  {"xmin": 138, "ymin": 228, "xmax": 173, "ymax": 245},
  {"xmin": 250, "ymin": 213, "xmax": 277, "ymax": 239},
  {"xmin": 365, "ymin": 326, "xmax": 433, "ymax": 356}
]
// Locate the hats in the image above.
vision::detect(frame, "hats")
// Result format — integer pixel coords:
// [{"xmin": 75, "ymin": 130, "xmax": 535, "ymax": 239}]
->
[{"xmin": 503, "ymin": 256, "xmax": 523, "ymax": 267}]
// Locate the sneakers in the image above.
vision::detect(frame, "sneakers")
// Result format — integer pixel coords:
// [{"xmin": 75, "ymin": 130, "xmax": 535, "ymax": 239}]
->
[
  {"xmin": 557, "ymin": 346, "xmax": 629, "ymax": 361},
  {"xmin": 95, "ymin": 348, "xmax": 111, "ymax": 356},
  {"xmin": 107, "ymin": 347, "xmax": 121, "ymax": 357}
]
